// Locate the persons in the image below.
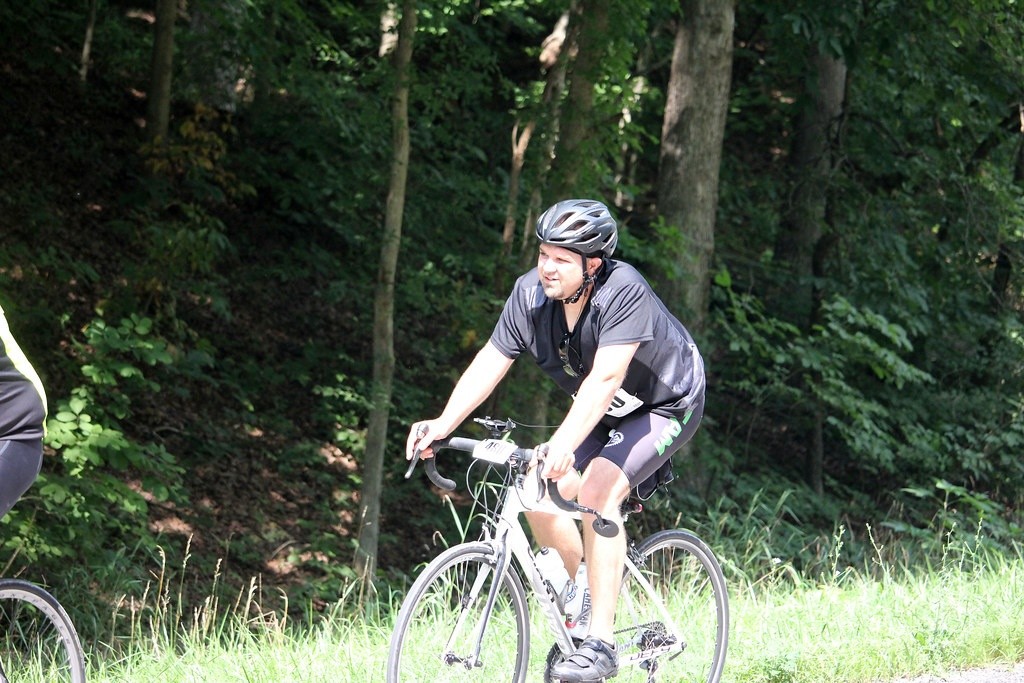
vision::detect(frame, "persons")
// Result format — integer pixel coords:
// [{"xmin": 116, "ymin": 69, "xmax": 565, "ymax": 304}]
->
[
  {"xmin": 406, "ymin": 199, "xmax": 707, "ymax": 682},
  {"xmin": 0, "ymin": 306, "xmax": 49, "ymax": 520}
]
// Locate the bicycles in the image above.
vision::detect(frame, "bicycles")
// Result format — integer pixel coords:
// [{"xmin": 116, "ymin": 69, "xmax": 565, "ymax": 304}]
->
[
  {"xmin": 386, "ymin": 422, "xmax": 729, "ymax": 683},
  {"xmin": 0, "ymin": 577, "xmax": 87, "ymax": 683}
]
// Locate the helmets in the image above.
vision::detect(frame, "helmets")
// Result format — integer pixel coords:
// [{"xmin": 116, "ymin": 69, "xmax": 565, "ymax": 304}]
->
[{"xmin": 535, "ymin": 198, "xmax": 619, "ymax": 258}]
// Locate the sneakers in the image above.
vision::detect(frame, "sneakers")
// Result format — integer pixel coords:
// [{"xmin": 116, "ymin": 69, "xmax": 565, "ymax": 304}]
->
[{"xmin": 549, "ymin": 636, "xmax": 619, "ymax": 683}]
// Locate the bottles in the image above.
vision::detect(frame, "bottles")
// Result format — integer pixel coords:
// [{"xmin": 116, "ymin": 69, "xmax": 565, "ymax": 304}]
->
[
  {"xmin": 564, "ymin": 556, "xmax": 591, "ymax": 641},
  {"xmin": 536, "ymin": 546, "xmax": 572, "ymax": 607}
]
